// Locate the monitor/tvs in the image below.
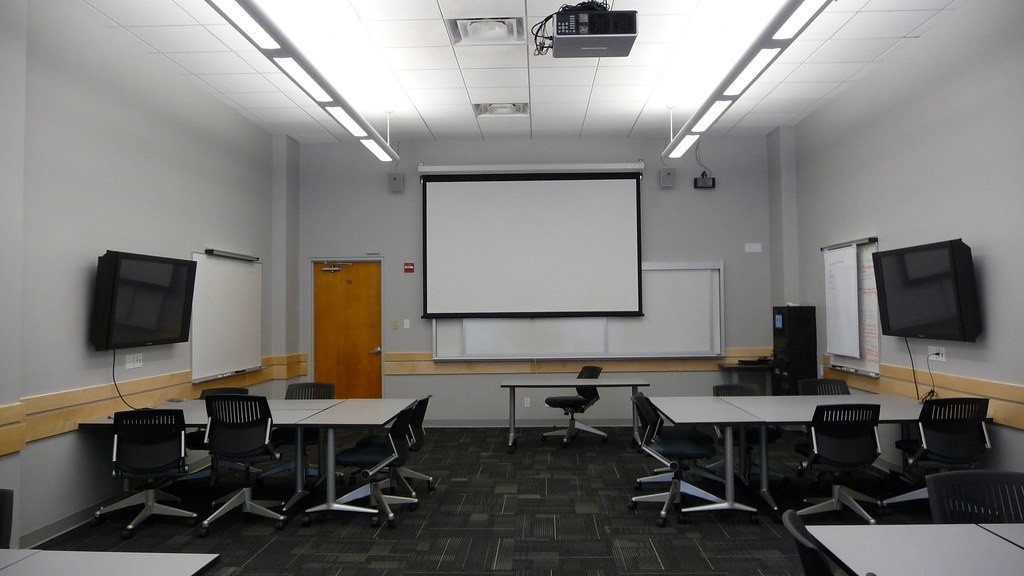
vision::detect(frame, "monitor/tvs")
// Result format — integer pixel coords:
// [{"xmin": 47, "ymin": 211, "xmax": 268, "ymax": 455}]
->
[
  {"xmin": 90, "ymin": 249, "xmax": 197, "ymax": 351},
  {"xmin": 872, "ymin": 238, "xmax": 983, "ymax": 342}
]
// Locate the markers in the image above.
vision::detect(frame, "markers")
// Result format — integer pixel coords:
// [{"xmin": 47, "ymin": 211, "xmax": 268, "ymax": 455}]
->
[
  {"xmin": 217, "ymin": 373, "xmax": 231, "ymax": 378},
  {"xmin": 870, "ymin": 371, "xmax": 879, "ymax": 376},
  {"xmin": 850, "ymin": 368, "xmax": 858, "ymax": 373}
]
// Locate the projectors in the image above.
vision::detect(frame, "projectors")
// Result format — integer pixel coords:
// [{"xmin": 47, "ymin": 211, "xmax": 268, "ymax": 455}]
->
[{"xmin": 552, "ymin": 11, "xmax": 637, "ymax": 57}]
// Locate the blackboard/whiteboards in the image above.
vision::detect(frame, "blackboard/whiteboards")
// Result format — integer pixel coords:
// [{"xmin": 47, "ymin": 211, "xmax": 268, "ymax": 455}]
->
[
  {"xmin": 190, "ymin": 251, "xmax": 266, "ymax": 384},
  {"xmin": 820, "ymin": 238, "xmax": 881, "ymax": 378}
]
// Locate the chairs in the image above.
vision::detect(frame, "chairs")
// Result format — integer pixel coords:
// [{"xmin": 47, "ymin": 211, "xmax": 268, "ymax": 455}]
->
[
  {"xmin": 95, "ymin": 409, "xmax": 199, "ymax": 542},
  {"xmin": 350, "ymin": 392, "xmax": 434, "ymax": 508},
  {"xmin": 268, "ymin": 382, "xmax": 334, "ymax": 505},
  {"xmin": 301, "ymin": 405, "xmax": 420, "ymax": 529},
  {"xmin": 796, "ymin": 377, "xmax": 873, "ymax": 491},
  {"xmin": 183, "ymin": 388, "xmax": 256, "ymax": 494},
  {"xmin": 538, "ymin": 365, "xmax": 607, "ymax": 447},
  {"xmin": 924, "ymin": 470, "xmax": 1024, "ymax": 524},
  {"xmin": 772, "ymin": 402, "xmax": 896, "ymax": 525},
  {"xmin": 892, "ymin": 397, "xmax": 988, "ymax": 523},
  {"xmin": 781, "ymin": 508, "xmax": 845, "ymax": 576},
  {"xmin": 198, "ymin": 393, "xmax": 297, "ymax": 539},
  {"xmin": 629, "ymin": 391, "xmax": 730, "ymax": 528},
  {"xmin": 711, "ymin": 381, "xmax": 796, "ymax": 500}
]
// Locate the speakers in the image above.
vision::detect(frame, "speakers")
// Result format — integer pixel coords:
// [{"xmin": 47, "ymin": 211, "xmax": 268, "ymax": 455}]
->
[
  {"xmin": 387, "ymin": 170, "xmax": 404, "ymax": 192},
  {"xmin": 660, "ymin": 167, "xmax": 675, "ymax": 187}
]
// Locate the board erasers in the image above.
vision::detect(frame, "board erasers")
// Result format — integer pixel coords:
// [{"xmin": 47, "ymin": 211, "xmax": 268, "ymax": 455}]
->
[
  {"xmin": 831, "ymin": 365, "xmax": 842, "ymax": 369},
  {"xmin": 234, "ymin": 369, "xmax": 246, "ymax": 374}
]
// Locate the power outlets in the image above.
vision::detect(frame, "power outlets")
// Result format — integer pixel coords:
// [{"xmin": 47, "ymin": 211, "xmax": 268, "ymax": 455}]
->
[{"xmin": 928, "ymin": 346, "xmax": 945, "ymax": 362}]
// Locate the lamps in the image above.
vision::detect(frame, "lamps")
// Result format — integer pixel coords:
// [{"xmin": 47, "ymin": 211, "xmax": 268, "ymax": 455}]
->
[
  {"xmin": 205, "ymin": 0, "xmax": 399, "ymax": 162},
  {"xmin": 661, "ymin": 0, "xmax": 835, "ymax": 166}
]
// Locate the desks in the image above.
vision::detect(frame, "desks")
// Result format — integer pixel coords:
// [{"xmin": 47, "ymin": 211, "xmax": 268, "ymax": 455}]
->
[{"xmin": 0, "ymin": 362, "xmax": 1024, "ymax": 576}]
